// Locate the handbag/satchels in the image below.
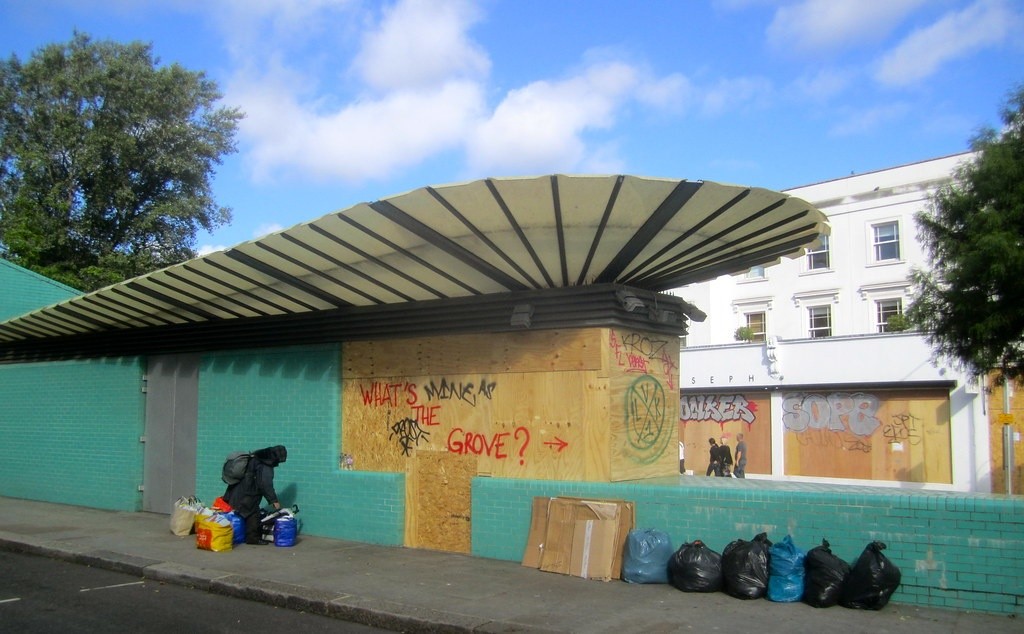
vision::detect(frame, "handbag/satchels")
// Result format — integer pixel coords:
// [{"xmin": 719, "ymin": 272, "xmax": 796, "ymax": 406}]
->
[
  {"xmin": 227, "ymin": 509, "xmax": 249, "ymax": 544},
  {"xmin": 798, "ymin": 537, "xmax": 851, "ymax": 607},
  {"xmin": 171, "ymin": 497, "xmax": 197, "ymax": 536},
  {"xmin": 717, "ymin": 529, "xmax": 773, "ymax": 599},
  {"xmin": 763, "ymin": 533, "xmax": 807, "ymax": 604},
  {"xmin": 623, "ymin": 525, "xmax": 675, "ymax": 585},
  {"xmin": 210, "ymin": 495, "xmax": 233, "ymax": 514},
  {"xmin": 192, "ymin": 495, "xmax": 213, "ymax": 534},
  {"xmin": 669, "ymin": 539, "xmax": 724, "ymax": 592},
  {"xmin": 274, "ymin": 510, "xmax": 300, "ymax": 547},
  {"xmin": 260, "ymin": 509, "xmax": 275, "ymax": 543},
  {"xmin": 196, "ymin": 514, "xmax": 234, "ymax": 551},
  {"xmin": 846, "ymin": 540, "xmax": 902, "ymax": 610}
]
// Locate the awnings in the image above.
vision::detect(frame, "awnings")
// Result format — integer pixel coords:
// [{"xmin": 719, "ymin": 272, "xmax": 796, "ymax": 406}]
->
[{"xmin": 0, "ymin": 172, "xmax": 831, "ymax": 352}]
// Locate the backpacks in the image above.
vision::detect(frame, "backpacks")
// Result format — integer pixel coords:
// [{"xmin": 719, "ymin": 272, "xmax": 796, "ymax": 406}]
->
[{"xmin": 218, "ymin": 449, "xmax": 261, "ymax": 488}]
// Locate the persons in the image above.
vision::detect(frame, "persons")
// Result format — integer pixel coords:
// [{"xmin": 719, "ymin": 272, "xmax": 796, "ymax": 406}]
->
[
  {"xmin": 678, "ymin": 441, "xmax": 685, "ymax": 474},
  {"xmin": 705, "ymin": 437, "xmax": 720, "ymax": 476},
  {"xmin": 733, "ymin": 433, "xmax": 747, "ymax": 478},
  {"xmin": 718, "ymin": 437, "xmax": 732, "ymax": 477},
  {"xmin": 224, "ymin": 445, "xmax": 287, "ymax": 544}
]
